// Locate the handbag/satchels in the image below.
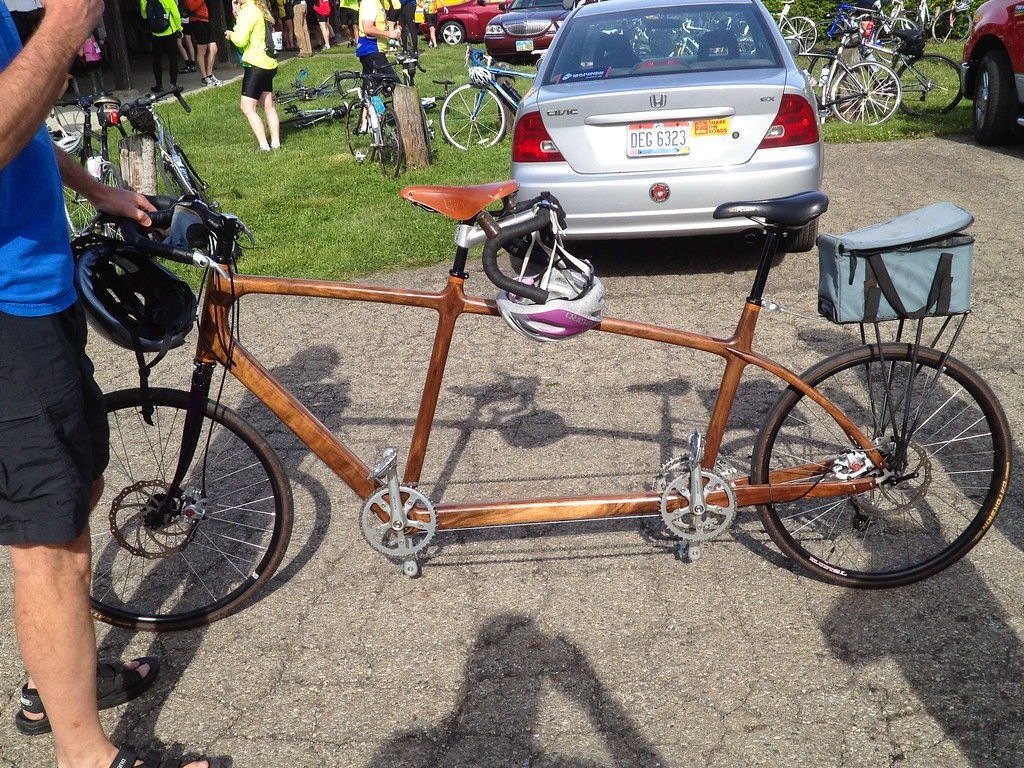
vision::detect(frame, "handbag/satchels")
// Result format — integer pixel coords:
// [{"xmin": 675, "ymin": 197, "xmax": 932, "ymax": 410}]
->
[{"xmin": 815, "ymin": 200, "xmax": 976, "ymax": 325}]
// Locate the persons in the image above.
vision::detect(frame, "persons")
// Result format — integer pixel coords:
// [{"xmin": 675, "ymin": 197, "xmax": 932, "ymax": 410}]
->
[
  {"xmin": 56, "ymin": 32, "xmax": 101, "ymax": 102},
  {"xmin": 383, "ymin": 0, "xmax": 449, "ymax": 56},
  {"xmin": 0, "ymin": 0, "xmax": 212, "ymax": 768},
  {"xmin": 267, "ymin": 0, "xmax": 360, "ymax": 59},
  {"xmin": 223, "ymin": 0, "xmax": 282, "ymax": 150},
  {"xmin": 139, "ymin": 0, "xmax": 186, "ymax": 94},
  {"xmin": 174, "ymin": 0, "xmax": 223, "ymax": 87},
  {"xmin": 355, "ymin": 0, "xmax": 402, "ymax": 94}
]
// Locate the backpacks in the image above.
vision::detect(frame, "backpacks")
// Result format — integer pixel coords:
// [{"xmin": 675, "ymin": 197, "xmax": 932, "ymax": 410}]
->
[
  {"xmin": 263, "ymin": 7, "xmax": 284, "ymax": 59},
  {"xmin": 146, "ymin": 0, "xmax": 171, "ymax": 33}
]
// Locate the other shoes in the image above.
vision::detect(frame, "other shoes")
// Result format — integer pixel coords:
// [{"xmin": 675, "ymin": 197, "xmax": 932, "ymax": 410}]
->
[
  {"xmin": 329, "ymin": 37, "xmax": 339, "ymax": 44},
  {"xmin": 346, "ymin": 40, "xmax": 359, "ymax": 48},
  {"xmin": 179, "ymin": 65, "xmax": 197, "ymax": 73},
  {"xmin": 150, "ymin": 86, "xmax": 163, "ymax": 92},
  {"xmin": 311, "ymin": 43, "xmax": 331, "ymax": 51},
  {"xmin": 169, "ymin": 82, "xmax": 178, "ymax": 90}
]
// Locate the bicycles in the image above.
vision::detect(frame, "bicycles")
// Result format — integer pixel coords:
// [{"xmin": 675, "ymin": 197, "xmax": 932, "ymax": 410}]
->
[
  {"xmin": 270, "ymin": 47, "xmax": 454, "ymax": 184},
  {"xmin": 439, "ymin": 44, "xmax": 547, "ymax": 151},
  {"xmin": 603, "ymin": 0, "xmax": 975, "ymax": 128},
  {"xmin": 84, "ymin": 180, "xmax": 1016, "ymax": 634}
]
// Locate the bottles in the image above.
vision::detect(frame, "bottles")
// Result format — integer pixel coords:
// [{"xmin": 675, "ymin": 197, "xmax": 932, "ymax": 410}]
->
[
  {"xmin": 369, "ymin": 89, "xmax": 385, "ymax": 114},
  {"xmin": 425, "ymin": 2, "xmax": 429, "ymax": 11},
  {"xmin": 368, "ymin": 106, "xmax": 378, "ymax": 131},
  {"xmin": 502, "ymin": 80, "xmax": 523, "ymax": 104},
  {"xmin": 859, "ymin": 46, "xmax": 881, "ymax": 72},
  {"xmin": 819, "ymin": 64, "xmax": 830, "ymax": 87},
  {"xmin": 802, "ymin": 67, "xmax": 817, "ymax": 87},
  {"xmin": 388, "ymin": 25, "xmax": 402, "ymax": 46}
]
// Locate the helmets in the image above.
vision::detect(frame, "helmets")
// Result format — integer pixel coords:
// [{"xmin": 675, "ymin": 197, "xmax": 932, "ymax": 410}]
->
[
  {"xmin": 69, "ymin": 233, "xmax": 199, "ymax": 356},
  {"xmin": 467, "ymin": 66, "xmax": 492, "ymax": 89},
  {"xmin": 495, "ymin": 254, "xmax": 606, "ymax": 343},
  {"xmin": 53, "ymin": 130, "xmax": 81, "ymax": 155}
]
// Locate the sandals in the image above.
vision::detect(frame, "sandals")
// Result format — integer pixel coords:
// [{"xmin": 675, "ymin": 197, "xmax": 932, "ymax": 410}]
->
[
  {"xmin": 15, "ymin": 656, "xmax": 161, "ymax": 737},
  {"xmin": 99, "ymin": 741, "xmax": 221, "ymax": 768}
]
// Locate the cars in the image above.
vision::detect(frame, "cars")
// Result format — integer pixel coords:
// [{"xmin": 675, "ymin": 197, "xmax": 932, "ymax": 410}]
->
[
  {"xmin": 417, "ymin": 0, "xmax": 513, "ymax": 46},
  {"xmin": 484, "ymin": 0, "xmax": 583, "ymax": 62},
  {"xmin": 41, "ymin": 82, "xmax": 220, "ymax": 254},
  {"xmin": 959, "ymin": 0, "xmax": 1024, "ymax": 146},
  {"xmin": 510, "ymin": 0, "xmax": 827, "ymax": 263}
]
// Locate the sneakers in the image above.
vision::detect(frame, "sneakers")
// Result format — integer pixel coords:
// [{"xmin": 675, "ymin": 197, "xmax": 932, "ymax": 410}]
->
[
  {"xmin": 207, "ymin": 74, "xmax": 222, "ymax": 85},
  {"xmin": 201, "ymin": 76, "xmax": 217, "ymax": 87}
]
